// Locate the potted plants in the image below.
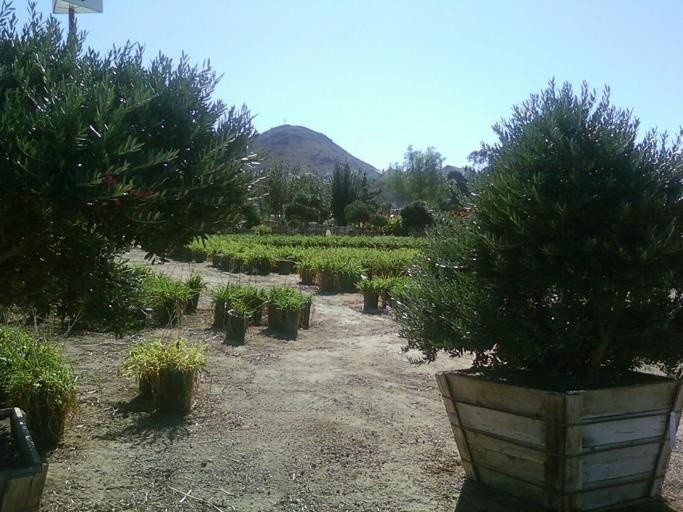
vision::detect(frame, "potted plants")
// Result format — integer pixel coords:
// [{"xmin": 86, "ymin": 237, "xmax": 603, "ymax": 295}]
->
[
  {"xmin": 122, "ymin": 341, "xmax": 205, "ymax": 393},
  {"xmin": 0, "ymin": 327, "xmax": 79, "ymax": 441},
  {"xmin": 177, "ymin": 249, "xmax": 390, "ymax": 340},
  {"xmin": 391, "ymin": 75, "xmax": 681, "ymax": 511}
]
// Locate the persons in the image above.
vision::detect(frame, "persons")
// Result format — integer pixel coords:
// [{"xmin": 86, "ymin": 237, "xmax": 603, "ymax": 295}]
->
[{"xmin": 324, "ymin": 225, "xmax": 332, "ymax": 237}]
